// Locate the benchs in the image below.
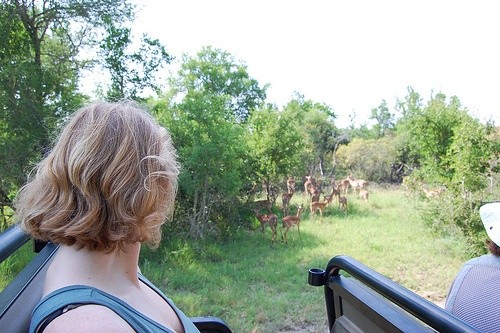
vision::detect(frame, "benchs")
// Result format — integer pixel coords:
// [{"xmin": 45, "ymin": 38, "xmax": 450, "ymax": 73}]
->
[
  {"xmin": 323, "ymin": 253, "xmax": 487, "ymax": 333},
  {"xmin": 0, "ymin": 222, "xmax": 233, "ymax": 333}
]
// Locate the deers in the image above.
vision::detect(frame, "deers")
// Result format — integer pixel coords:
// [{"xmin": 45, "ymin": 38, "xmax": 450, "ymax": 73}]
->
[
  {"xmin": 281, "ymin": 203, "xmax": 303, "ymax": 245},
  {"xmin": 246, "ymin": 175, "xmax": 370, "ymax": 246}
]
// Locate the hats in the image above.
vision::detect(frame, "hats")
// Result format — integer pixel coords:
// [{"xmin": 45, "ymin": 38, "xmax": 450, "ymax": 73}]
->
[{"xmin": 480, "ymin": 203, "xmax": 500, "ymax": 247}]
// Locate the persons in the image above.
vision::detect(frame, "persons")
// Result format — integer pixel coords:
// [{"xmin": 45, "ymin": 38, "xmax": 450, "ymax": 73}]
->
[
  {"xmin": 13, "ymin": 99, "xmax": 203, "ymax": 333},
  {"xmin": 444, "ymin": 202, "xmax": 500, "ymax": 333}
]
